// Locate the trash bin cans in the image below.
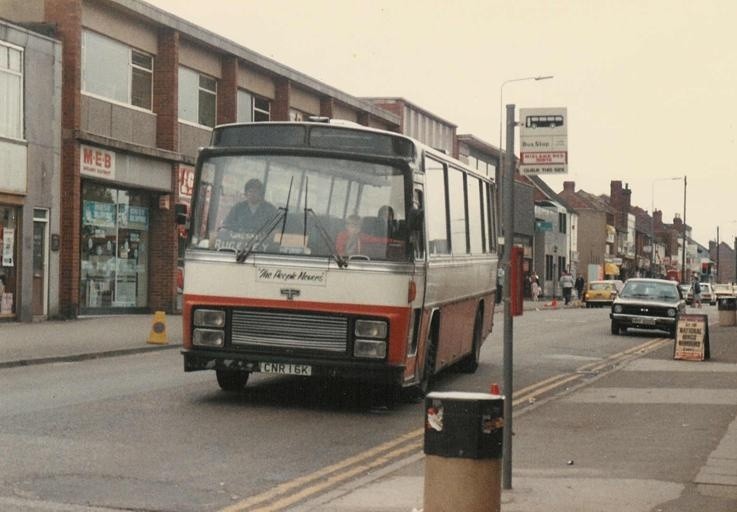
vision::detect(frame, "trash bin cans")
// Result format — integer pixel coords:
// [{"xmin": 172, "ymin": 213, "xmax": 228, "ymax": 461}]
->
[
  {"xmin": 718, "ymin": 298, "xmax": 737, "ymax": 325},
  {"xmin": 425, "ymin": 391, "xmax": 505, "ymax": 512}
]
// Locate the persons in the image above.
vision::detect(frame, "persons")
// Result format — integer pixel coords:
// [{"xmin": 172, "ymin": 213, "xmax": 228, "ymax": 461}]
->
[
  {"xmin": 496, "ymin": 264, "xmax": 505, "ymax": 302},
  {"xmin": 336, "ymin": 205, "xmax": 405, "ymax": 257},
  {"xmin": 222, "ymin": 179, "xmax": 279, "ymax": 241},
  {"xmin": 529, "ymin": 270, "xmax": 584, "ymax": 305},
  {"xmin": 690, "ymin": 275, "xmax": 702, "ymax": 307}
]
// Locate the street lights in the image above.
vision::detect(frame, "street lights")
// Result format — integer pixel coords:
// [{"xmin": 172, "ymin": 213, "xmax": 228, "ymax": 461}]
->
[{"xmin": 499, "ymin": 73, "xmax": 555, "ymax": 258}]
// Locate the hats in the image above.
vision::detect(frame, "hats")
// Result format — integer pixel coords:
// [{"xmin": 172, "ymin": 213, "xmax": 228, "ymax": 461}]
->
[{"xmin": 246, "ymin": 178, "xmax": 263, "ymax": 192}]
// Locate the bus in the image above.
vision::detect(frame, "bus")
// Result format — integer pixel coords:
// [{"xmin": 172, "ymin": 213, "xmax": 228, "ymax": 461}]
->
[
  {"xmin": 525, "ymin": 115, "xmax": 564, "ymax": 129},
  {"xmin": 175, "ymin": 115, "xmax": 500, "ymax": 403}
]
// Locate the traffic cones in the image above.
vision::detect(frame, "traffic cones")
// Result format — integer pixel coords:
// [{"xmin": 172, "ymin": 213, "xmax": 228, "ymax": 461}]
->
[{"xmin": 146, "ymin": 310, "xmax": 171, "ymax": 344}]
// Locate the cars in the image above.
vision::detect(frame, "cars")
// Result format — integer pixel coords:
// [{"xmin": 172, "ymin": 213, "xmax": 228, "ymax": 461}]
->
[{"xmin": 583, "ymin": 276, "xmax": 737, "ymax": 339}]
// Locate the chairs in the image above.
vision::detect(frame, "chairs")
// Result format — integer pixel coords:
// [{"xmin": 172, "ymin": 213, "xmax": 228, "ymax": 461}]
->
[{"xmin": 283, "ymin": 213, "xmax": 410, "ymax": 261}]
